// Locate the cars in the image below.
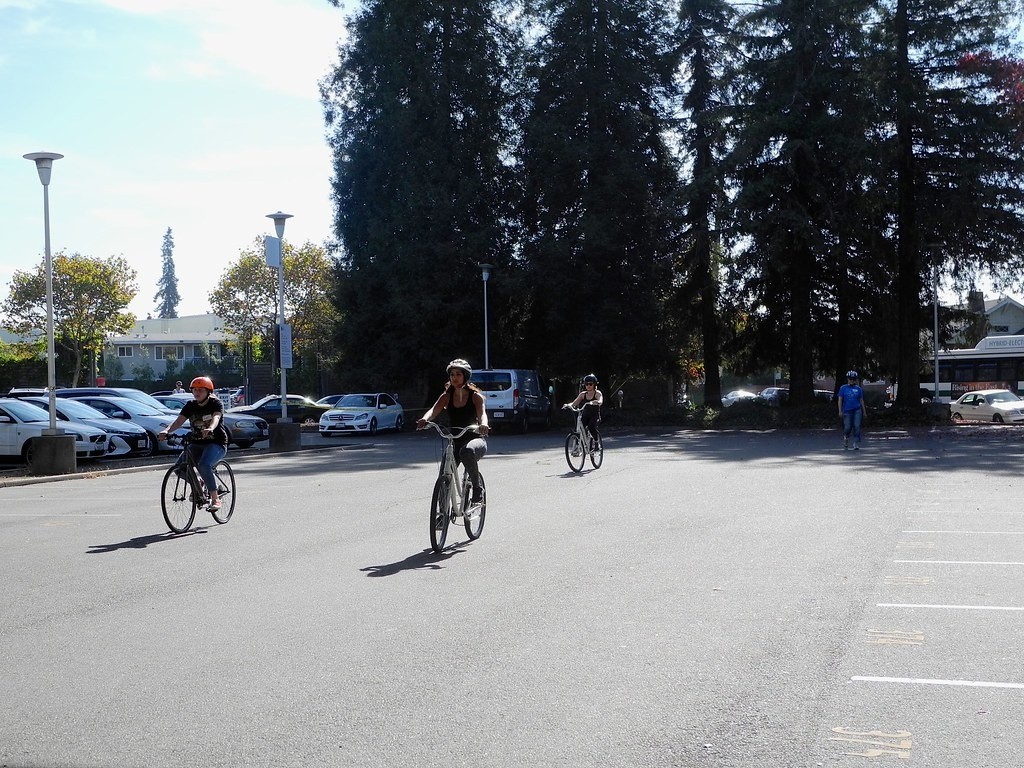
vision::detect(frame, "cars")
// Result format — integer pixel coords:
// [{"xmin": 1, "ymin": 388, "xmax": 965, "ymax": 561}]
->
[
  {"xmin": 814, "ymin": 389, "xmax": 834, "ymax": 401},
  {"xmin": 214, "ymin": 385, "xmax": 246, "ymax": 409},
  {"xmin": 721, "ymin": 390, "xmax": 758, "ymax": 408},
  {"xmin": 314, "ymin": 394, "xmax": 369, "ymax": 407},
  {"xmin": 319, "ymin": 392, "xmax": 406, "ymax": 438},
  {"xmin": 5, "ymin": 387, "xmax": 191, "ymax": 428},
  {"xmin": 151, "ymin": 395, "xmax": 270, "ymax": 449},
  {"xmin": 40, "ymin": 395, "xmax": 192, "ymax": 457},
  {"xmin": 224, "ymin": 394, "xmax": 335, "ymax": 424},
  {"xmin": 756, "ymin": 387, "xmax": 790, "ymax": 401},
  {"xmin": 947, "ymin": 388, "xmax": 1024, "ymax": 425},
  {"xmin": 16, "ymin": 396, "xmax": 150, "ymax": 459},
  {"xmin": 0, "ymin": 396, "xmax": 109, "ymax": 469}
]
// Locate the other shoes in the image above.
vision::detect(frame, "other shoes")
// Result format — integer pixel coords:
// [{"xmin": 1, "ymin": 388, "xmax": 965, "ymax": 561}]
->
[
  {"xmin": 852, "ymin": 443, "xmax": 859, "ymax": 450},
  {"xmin": 472, "ymin": 486, "xmax": 485, "ymax": 503},
  {"xmin": 843, "ymin": 438, "xmax": 849, "ymax": 447},
  {"xmin": 571, "ymin": 447, "xmax": 582, "ymax": 454},
  {"xmin": 436, "ymin": 516, "xmax": 444, "ymax": 529},
  {"xmin": 594, "ymin": 442, "xmax": 599, "ymax": 450}
]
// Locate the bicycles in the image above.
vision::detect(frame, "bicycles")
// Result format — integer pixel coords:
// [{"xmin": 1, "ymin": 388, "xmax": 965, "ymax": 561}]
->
[
  {"xmin": 157, "ymin": 428, "xmax": 237, "ymax": 534},
  {"xmin": 416, "ymin": 420, "xmax": 486, "ymax": 552},
  {"xmin": 561, "ymin": 402, "xmax": 604, "ymax": 473}
]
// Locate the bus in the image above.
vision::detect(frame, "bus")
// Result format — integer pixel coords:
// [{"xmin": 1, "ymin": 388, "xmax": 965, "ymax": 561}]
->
[{"xmin": 885, "ymin": 335, "xmax": 1024, "ymax": 412}]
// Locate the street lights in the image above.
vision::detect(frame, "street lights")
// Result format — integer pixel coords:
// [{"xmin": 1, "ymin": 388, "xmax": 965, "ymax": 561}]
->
[
  {"xmin": 266, "ymin": 210, "xmax": 295, "ymax": 421},
  {"xmin": 924, "ymin": 241, "xmax": 947, "ymax": 402},
  {"xmin": 23, "ymin": 152, "xmax": 66, "ymax": 436},
  {"xmin": 477, "ymin": 264, "xmax": 495, "ymax": 371}
]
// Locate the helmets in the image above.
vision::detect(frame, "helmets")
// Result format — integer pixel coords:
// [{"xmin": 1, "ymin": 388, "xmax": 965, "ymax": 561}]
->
[
  {"xmin": 584, "ymin": 374, "xmax": 597, "ymax": 384},
  {"xmin": 846, "ymin": 370, "xmax": 858, "ymax": 377},
  {"xmin": 190, "ymin": 377, "xmax": 214, "ymax": 390},
  {"xmin": 446, "ymin": 359, "xmax": 472, "ymax": 378}
]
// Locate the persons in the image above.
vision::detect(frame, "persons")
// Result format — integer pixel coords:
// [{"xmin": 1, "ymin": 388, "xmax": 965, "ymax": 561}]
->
[
  {"xmin": 172, "ymin": 381, "xmax": 186, "ymax": 393},
  {"xmin": 563, "ymin": 374, "xmax": 604, "ymax": 454},
  {"xmin": 158, "ymin": 378, "xmax": 228, "ymax": 511},
  {"xmin": 415, "ymin": 358, "xmax": 489, "ymax": 527},
  {"xmin": 838, "ymin": 371, "xmax": 867, "ymax": 450}
]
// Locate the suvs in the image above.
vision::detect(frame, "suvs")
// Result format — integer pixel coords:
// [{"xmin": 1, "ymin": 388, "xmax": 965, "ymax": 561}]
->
[{"xmin": 468, "ymin": 368, "xmax": 553, "ymax": 435}]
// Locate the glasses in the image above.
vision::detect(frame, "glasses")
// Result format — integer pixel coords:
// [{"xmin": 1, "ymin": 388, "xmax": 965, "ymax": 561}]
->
[
  {"xmin": 848, "ymin": 377, "xmax": 856, "ymax": 381},
  {"xmin": 585, "ymin": 382, "xmax": 593, "ymax": 386},
  {"xmin": 191, "ymin": 388, "xmax": 207, "ymax": 393},
  {"xmin": 177, "ymin": 384, "xmax": 182, "ymax": 386}
]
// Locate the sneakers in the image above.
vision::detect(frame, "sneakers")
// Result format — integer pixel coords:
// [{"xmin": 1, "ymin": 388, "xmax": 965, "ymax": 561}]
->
[
  {"xmin": 189, "ymin": 478, "xmax": 204, "ymax": 501},
  {"xmin": 210, "ymin": 499, "xmax": 221, "ymax": 510}
]
